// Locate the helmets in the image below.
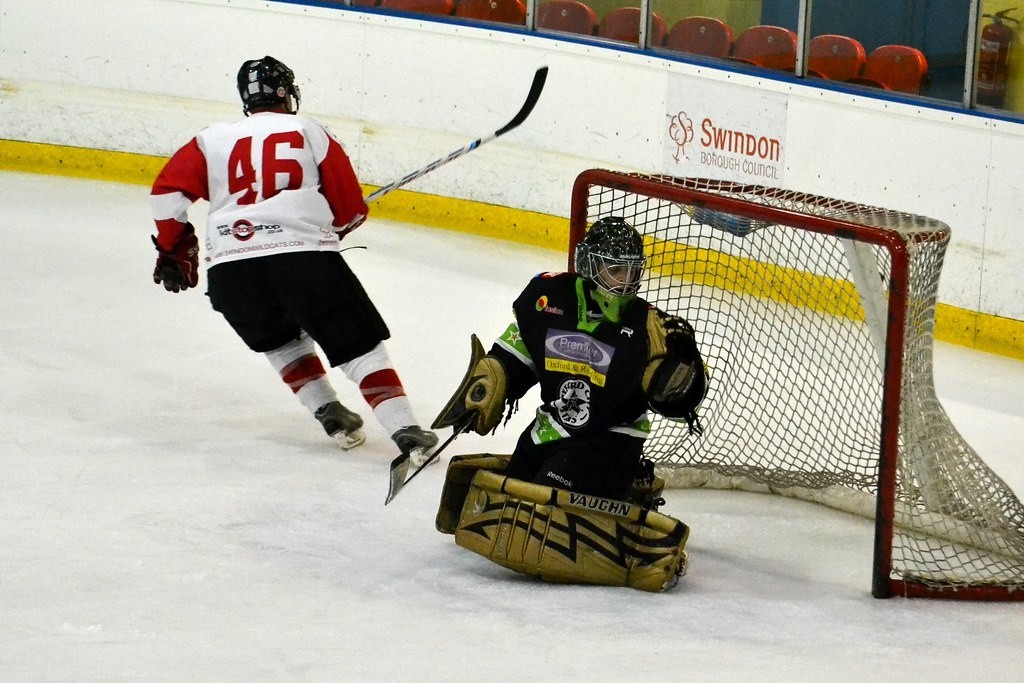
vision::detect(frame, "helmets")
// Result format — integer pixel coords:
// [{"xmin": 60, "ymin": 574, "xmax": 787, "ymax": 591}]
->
[
  {"xmin": 237, "ymin": 56, "xmax": 297, "ymax": 116},
  {"xmin": 575, "ymin": 216, "xmax": 647, "ymax": 322}
]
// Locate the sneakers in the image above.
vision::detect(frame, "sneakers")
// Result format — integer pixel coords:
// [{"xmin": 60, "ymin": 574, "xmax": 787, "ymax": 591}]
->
[
  {"xmin": 314, "ymin": 401, "xmax": 365, "ymax": 451},
  {"xmin": 392, "ymin": 426, "xmax": 440, "ymax": 469}
]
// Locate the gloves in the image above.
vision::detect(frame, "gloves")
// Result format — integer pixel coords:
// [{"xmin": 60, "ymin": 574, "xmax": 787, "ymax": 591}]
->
[{"xmin": 151, "ymin": 221, "xmax": 199, "ymax": 292}]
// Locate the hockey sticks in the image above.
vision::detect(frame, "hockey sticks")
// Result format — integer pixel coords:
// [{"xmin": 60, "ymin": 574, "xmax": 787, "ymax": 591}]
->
[
  {"xmin": 359, "ymin": 65, "xmax": 550, "ymax": 206},
  {"xmin": 384, "ymin": 414, "xmax": 475, "ymax": 507}
]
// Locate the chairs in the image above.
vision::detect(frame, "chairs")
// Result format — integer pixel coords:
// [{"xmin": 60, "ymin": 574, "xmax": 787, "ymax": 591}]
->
[{"xmin": 345, "ymin": 0, "xmax": 935, "ymax": 97}]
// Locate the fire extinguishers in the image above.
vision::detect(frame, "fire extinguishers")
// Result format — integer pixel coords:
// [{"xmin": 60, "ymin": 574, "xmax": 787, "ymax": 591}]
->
[{"xmin": 974, "ymin": 8, "xmax": 1019, "ymax": 111}]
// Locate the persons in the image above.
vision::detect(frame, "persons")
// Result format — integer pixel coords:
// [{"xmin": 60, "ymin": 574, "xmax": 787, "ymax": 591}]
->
[
  {"xmin": 452, "ymin": 217, "xmax": 711, "ymax": 504},
  {"xmin": 150, "ymin": 55, "xmax": 437, "ymax": 457}
]
